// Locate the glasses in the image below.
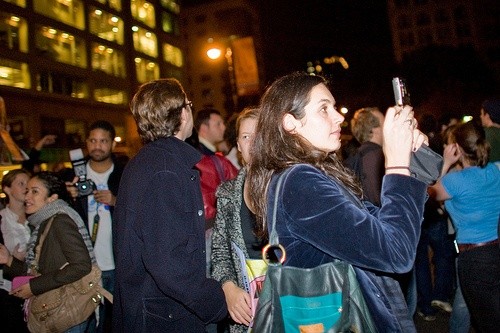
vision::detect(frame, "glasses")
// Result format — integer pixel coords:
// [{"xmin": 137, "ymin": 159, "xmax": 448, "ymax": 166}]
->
[{"xmin": 182, "ymin": 100, "xmax": 193, "ymax": 108}]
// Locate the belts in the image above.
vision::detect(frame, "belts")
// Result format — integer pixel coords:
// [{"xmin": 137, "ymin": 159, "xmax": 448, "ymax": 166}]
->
[{"xmin": 454, "ymin": 237, "xmax": 500, "ymax": 253}]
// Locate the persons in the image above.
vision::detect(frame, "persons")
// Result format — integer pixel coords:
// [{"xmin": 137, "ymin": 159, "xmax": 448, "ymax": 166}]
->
[
  {"xmin": 349, "ymin": 99, "xmax": 500, "ymax": 333},
  {"xmin": 0, "ymin": 95, "xmax": 124, "ymax": 333},
  {"xmin": 112, "ymin": 78, "xmax": 228, "ymax": 333},
  {"xmin": 194, "ymin": 108, "xmax": 274, "ymax": 333},
  {"xmin": 250, "ymin": 71, "xmax": 429, "ymax": 333}
]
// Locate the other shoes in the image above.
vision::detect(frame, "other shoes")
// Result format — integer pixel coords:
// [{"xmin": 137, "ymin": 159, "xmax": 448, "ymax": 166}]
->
[
  {"xmin": 431, "ymin": 296, "xmax": 453, "ymax": 312},
  {"xmin": 419, "ymin": 309, "xmax": 435, "ymax": 321}
]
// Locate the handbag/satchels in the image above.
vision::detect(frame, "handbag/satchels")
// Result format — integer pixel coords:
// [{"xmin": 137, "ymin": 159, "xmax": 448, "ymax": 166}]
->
[
  {"xmin": 25, "ymin": 215, "xmax": 114, "ymax": 333},
  {"xmin": 250, "ymin": 163, "xmax": 376, "ymax": 333}
]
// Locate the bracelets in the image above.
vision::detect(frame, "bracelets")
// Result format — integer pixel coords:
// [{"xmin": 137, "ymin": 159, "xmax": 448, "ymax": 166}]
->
[{"xmin": 384, "ymin": 166, "xmax": 409, "ymax": 170}]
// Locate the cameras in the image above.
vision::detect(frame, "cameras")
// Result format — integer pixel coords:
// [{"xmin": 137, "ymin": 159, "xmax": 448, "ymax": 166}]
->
[
  {"xmin": 68, "ymin": 148, "xmax": 98, "ymax": 197},
  {"xmin": 392, "ymin": 77, "xmax": 411, "ymax": 106}
]
